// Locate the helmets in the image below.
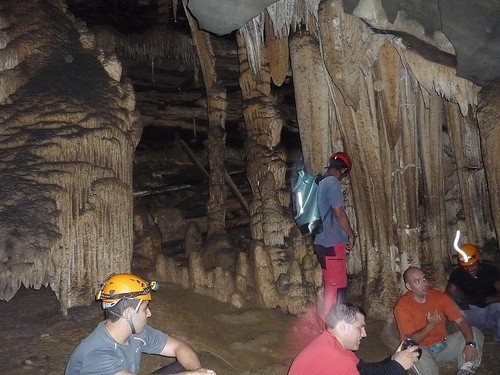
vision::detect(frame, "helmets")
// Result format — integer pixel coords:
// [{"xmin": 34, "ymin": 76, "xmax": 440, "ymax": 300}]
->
[
  {"xmin": 330, "ymin": 151, "xmax": 352, "ymax": 172},
  {"xmin": 101, "ymin": 273, "xmax": 151, "ymax": 303},
  {"xmin": 457, "ymin": 244, "xmax": 481, "ymax": 266}
]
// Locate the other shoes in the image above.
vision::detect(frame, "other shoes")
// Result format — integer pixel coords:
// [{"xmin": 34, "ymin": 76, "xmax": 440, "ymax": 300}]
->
[{"xmin": 457, "ymin": 362, "xmax": 475, "ymax": 375}]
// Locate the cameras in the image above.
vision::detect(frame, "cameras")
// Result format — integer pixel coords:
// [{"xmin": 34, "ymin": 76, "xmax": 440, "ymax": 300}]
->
[{"xmin": 402, "ymin": 339, "xmax": 422, "ymax": 360}]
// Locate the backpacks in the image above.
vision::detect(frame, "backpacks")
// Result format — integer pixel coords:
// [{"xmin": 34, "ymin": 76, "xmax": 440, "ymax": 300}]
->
[{"xmin": 291, "ymin": 169, "xmax": 333, "ymax": 236}]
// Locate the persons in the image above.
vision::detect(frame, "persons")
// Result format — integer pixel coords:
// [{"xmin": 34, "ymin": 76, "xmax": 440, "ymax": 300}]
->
[
  {"xmin": 288, "ymin": 301, "xmax": 419, "ymax": 375},
  {"xmin": 65, "ymin": 274, "xmax": 217, "ymax": 375},
  {"xmin": 443, "ymin": 244, "xmax": 500, "ymax": 343},
  {"xmin": 394, "ymin": 267, "xmax": 484, "ymax": 375},
  {"xmin": 312, "ymin": 152, "xmax": 356, "ymax": 330}
]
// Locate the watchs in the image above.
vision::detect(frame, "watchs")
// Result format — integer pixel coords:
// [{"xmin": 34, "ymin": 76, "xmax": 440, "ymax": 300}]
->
[{"xmin": 466, "ymin": 340, "xmax": 477, "ymax": 347}]
